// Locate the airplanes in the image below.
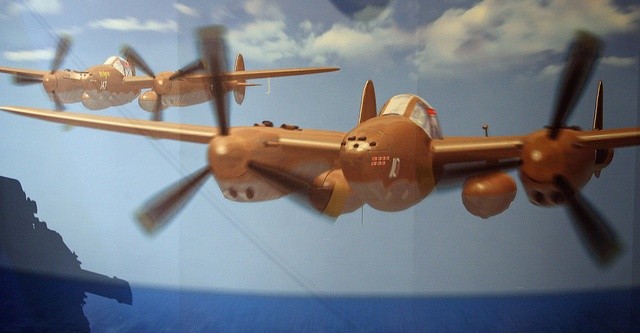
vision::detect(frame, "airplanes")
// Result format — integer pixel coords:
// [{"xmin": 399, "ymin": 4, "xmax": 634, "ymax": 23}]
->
[
  {"xmin": 0, "ymin": 24, "xmax": 640, "ymax": 267},
  {"xmin": 0, "ymin": 33, "xmax": 340, "ymax": 121}
]
